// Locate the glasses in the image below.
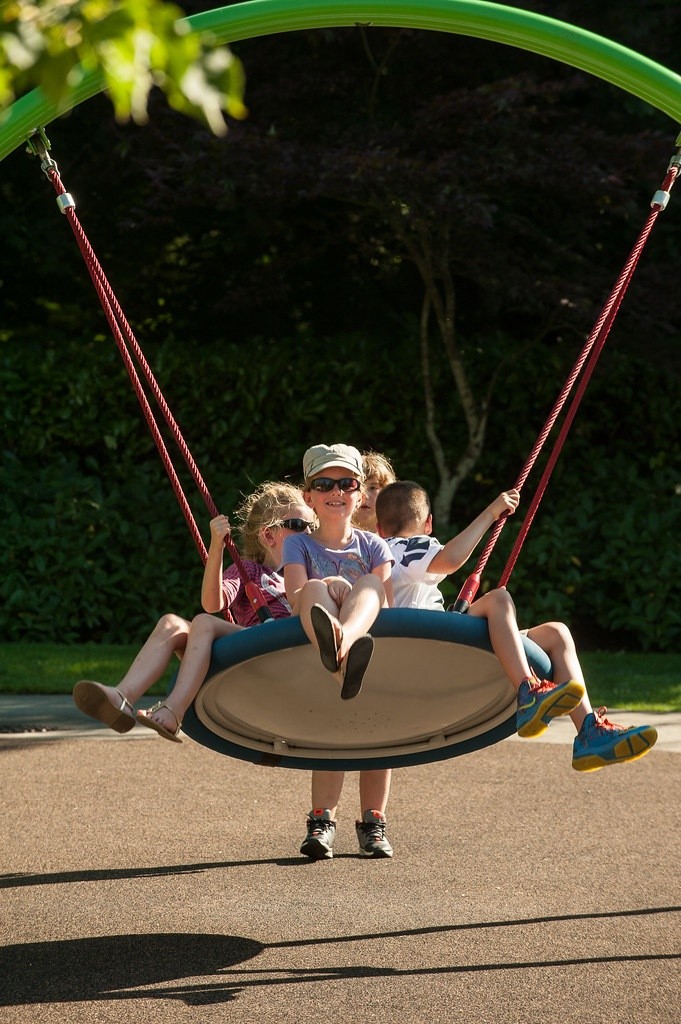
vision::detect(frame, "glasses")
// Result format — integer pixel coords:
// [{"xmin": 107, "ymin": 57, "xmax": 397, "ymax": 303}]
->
[
  {"xmin": 270, "ymin": 519, "xmax": 317, "ymax": 533},
  {"xmin": 307, "ymin": 477, "xmax": 360, "ymax": 492}
]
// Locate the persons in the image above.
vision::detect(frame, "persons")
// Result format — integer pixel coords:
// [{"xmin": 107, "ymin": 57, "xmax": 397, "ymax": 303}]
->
[
  {"xmin": 282, "ymin": 443, "xmax": 396, "ymax": 699},
  {"xmin": 72, "ymin": 481, "xmax": 322, "ymax": 745},
  {"xmin": 374, "ymin": 482, "xmax": 658, "ymax": 773},
  {"xmin": 300, "ymin": 451, "xmax": 397, "ymax": 859}
]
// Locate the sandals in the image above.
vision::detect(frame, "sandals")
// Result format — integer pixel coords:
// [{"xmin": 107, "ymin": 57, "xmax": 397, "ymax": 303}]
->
[
  {"xmin": 135, "ymin": 701, "xmax": 183, "ymax": 744},
  {"xmin": 73, "ymin": 680, "xmax": 135, "ymax": 734}
]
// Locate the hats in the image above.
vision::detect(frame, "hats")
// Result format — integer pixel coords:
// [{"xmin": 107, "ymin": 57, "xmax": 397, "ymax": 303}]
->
[{"xmin": 302, "ymin": 443, "xmax": 364, "ymax": 484}]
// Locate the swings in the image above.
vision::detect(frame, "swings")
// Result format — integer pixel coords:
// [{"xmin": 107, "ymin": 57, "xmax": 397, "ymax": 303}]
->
[{"xmin": 26, "ymin": 119, "xmax": 679, "ymax": 773}]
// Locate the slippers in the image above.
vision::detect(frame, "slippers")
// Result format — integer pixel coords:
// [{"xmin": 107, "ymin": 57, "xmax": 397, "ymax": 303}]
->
[
  {"xmin": 310, "ymin": 604, "xmax": 343, "ymax": 672},
  {"xmin": 341, "ymin": 635, "xmax": 374, "ymax": 701}
]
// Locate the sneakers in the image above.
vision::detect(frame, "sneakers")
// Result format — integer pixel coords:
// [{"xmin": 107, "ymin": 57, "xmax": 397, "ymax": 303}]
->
[
  {"xmin": 356, "ymin": 809, "xmax": 393, "ymax": 858},
  {"xmin": 516, "ymin": 666, "xmax": 584, "ymax": 737},
  {"xmin": 572, "ymin": 705, "xmax": 658, "ymax": 772},
  {"xmin": 300, "ymin": 808, "xmax": 336, "ymax": 859}
]
